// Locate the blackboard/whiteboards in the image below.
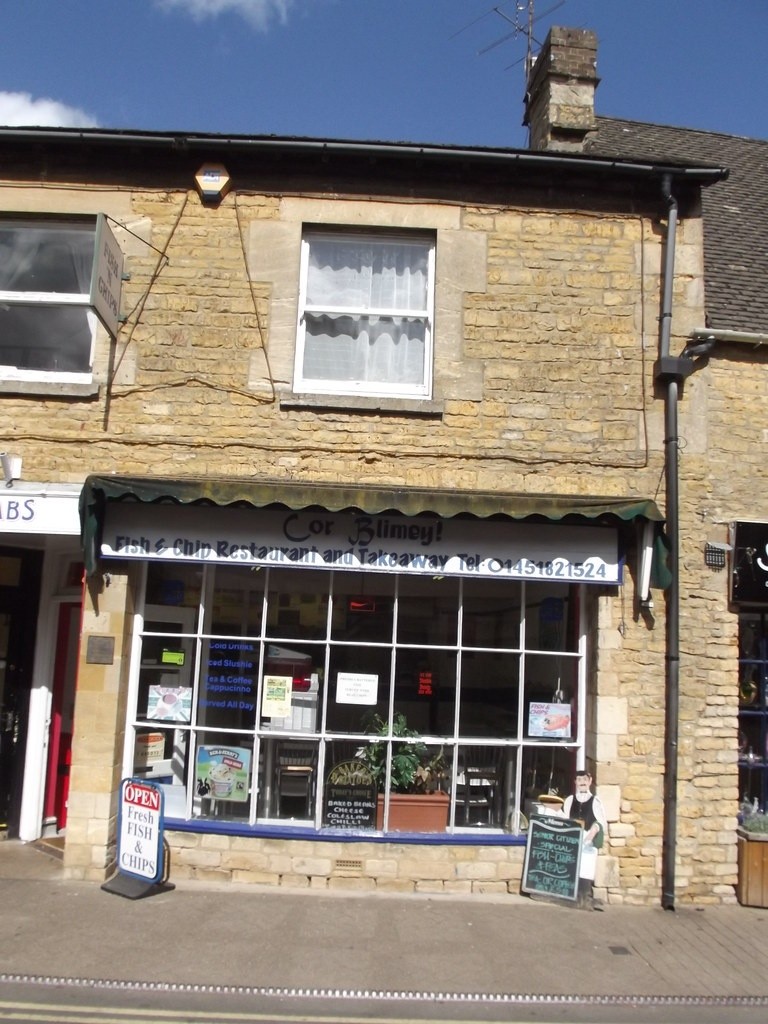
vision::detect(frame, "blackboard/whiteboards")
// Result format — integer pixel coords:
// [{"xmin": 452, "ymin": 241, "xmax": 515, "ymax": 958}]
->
[
  {"xmin": 522, "ymin": 813, "xmax": 584, "ymax": 901},
  {"xmin": 323, "ymin": 761, "xmax": 378, "ymax": 829}
]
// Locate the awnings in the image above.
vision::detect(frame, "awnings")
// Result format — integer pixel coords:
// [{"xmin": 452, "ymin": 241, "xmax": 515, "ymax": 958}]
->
[{"xmin": 77, "ymin": 467, "xmax": 674, "ymax": 610}]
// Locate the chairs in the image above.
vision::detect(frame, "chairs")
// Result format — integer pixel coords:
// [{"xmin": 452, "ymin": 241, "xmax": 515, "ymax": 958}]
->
[
  {"xmin": 456, "ymin": 745, "xmax": 504, "ymax": 828},
  {"xmin": 274, "ymin": 740, "xmax": 317, "ymax": 820}
]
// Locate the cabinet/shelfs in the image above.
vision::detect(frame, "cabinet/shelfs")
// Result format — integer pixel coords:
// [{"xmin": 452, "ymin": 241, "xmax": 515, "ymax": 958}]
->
[{"xmin": 738, "ymin": 659, "xmax": 768, "ymax": 813}]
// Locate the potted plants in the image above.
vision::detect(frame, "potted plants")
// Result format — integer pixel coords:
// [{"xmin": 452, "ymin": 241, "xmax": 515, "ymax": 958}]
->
[
  {"xmin": 354, "ymin": 711, "xmax": 451, "ymax": 831},
  {"xmin": 737, "ymin": 813, "xmax": 768, "ymax": 909}
]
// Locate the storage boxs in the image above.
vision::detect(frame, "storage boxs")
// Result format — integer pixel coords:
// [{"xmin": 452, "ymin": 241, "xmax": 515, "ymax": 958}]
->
[{"xmin": 524, "ymin": 798, "xmax": 564, "ymax": 820}]
